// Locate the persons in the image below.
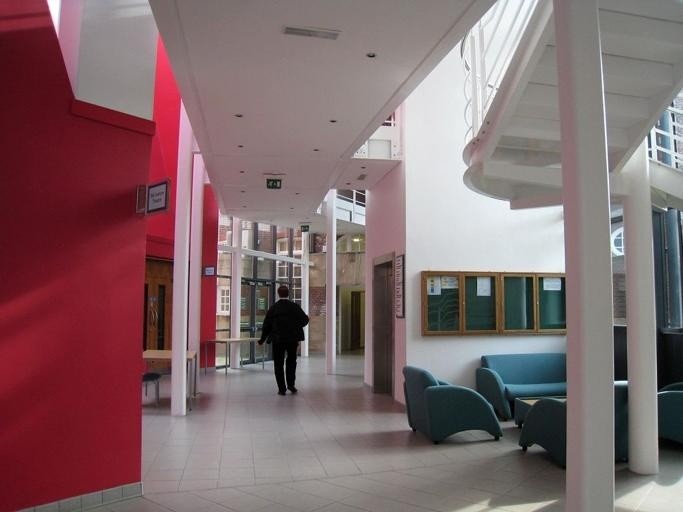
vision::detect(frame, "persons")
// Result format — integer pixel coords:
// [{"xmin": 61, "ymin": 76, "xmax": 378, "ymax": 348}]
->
[{"xmin": 256, "ymin": 285, "xmax": 310, "ymax": 396}]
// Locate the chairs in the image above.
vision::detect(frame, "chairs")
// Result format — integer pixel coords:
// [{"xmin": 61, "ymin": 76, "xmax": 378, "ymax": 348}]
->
[
  {"xmin": 400, "ymin": 366, "xmax": 502, "ymax": 443},
  {"xmin": 514, "ymin": 380, "xmax": 683, "ymax": 468}
]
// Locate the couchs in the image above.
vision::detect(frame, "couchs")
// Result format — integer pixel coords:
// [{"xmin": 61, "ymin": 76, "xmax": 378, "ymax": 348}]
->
[{"xmin": 475, "ymin": 352, "xmax": 565, "ymax": 421}]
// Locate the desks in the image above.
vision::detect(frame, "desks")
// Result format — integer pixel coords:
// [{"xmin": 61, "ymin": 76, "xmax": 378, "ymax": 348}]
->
[
  {"xmin": 139, "ymin": 350, "xmax": 198, "ymax": 411},
  {"xmin": 202, "ymin": 337, "xmax": 267, "ymax": 376}
]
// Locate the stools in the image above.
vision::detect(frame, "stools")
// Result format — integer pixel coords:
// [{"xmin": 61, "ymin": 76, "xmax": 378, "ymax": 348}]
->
[{"xmin": 142, "ymin": 372, "xmax": 162, "ymax": 403}]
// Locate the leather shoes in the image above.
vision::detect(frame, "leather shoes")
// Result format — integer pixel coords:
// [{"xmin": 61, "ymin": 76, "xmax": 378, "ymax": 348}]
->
[
  {"xmin": 288, "ymin": 386, "xmax": 297, "ymax": 392},
  {"xmin": 277, "ymin": 391, "xmax": 286, "ymax": 396}
]
